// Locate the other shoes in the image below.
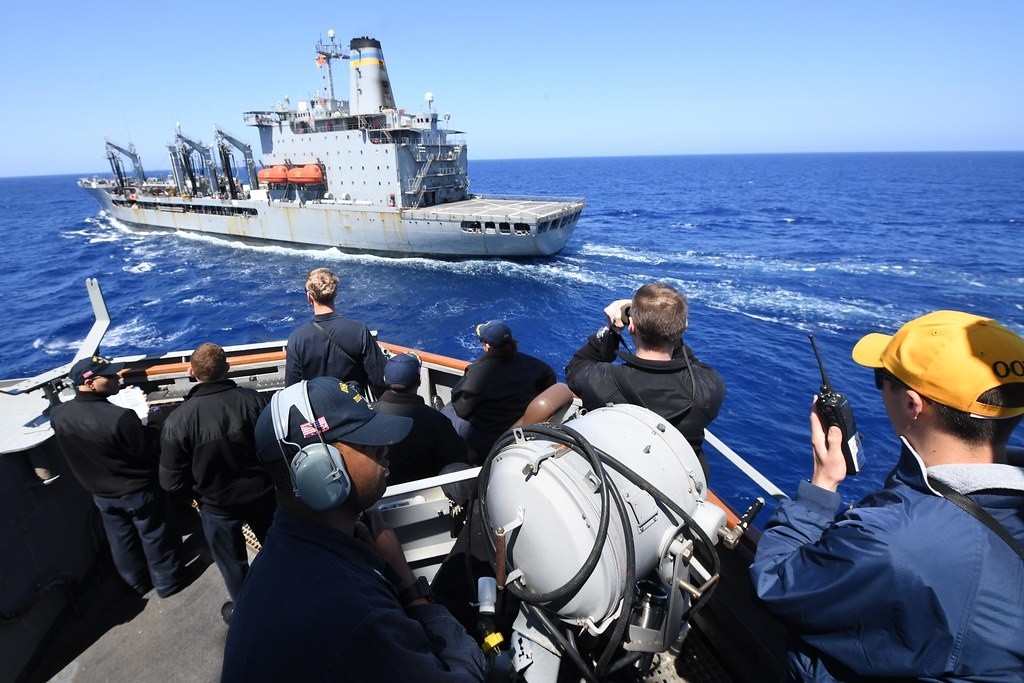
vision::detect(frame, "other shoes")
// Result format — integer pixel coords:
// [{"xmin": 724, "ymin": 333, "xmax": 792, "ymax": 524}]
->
[
  {"xmin": 138, "ymin": 584, "xmax": 154, "ymax": 594},
  {"xmin": 157, "ymin": 577, "xmax": 195, "ymax": 598}
]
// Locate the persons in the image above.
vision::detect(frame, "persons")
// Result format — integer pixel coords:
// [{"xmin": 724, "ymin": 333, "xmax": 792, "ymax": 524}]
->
[
  {"xmin": 563, "ymin": 284, "xmax": 728, "ymax": 496},
  {"xmin": 368, "ymin": 352, "xmax": 474, "ymax": 487},
  {"xmin": 158, "ymin": 342, "xmax": 277, "ymax": 628},
  {"xmin": 745, "ymin": 308, "xmax": 1024, "ymax": 683},
  {"xmin": 284, "ymin": 268, "xmax": 389, "ymax": 403},
  {"xmin": 48, "ymin": 355, "xmax": 206, "ymax": 599},
  {"xmin": 218, "ymin": 376, "xmax": 488, "ymax": 683},
  {"xmin": 440, "ymin": 319, "xmax": 558, "ymax": 453}
]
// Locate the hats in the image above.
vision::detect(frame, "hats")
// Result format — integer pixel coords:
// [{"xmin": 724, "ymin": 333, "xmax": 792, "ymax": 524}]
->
[
  {"xmin": 68, "ymin": 355, "xmax": 126, "ymax": 384},
  {"xmin": 382, "ymin": 351, "xmax": 423, "ymax": 388},
  {"xmin": 476, "ymin": 320, "xmax": 512, "ymax": 349},
  {"xmin": 253, "ymin": 376, "xmax": 414, "ymax": 465},
  {"xmin": 851, "ymin": 310, "xmax": 1024, "ymax": 419}
]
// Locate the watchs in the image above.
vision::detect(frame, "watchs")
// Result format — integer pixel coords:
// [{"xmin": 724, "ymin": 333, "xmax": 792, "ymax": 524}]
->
[{"xmin": 399, "ymin": 574, "xmax": 435, "ymax": 605}]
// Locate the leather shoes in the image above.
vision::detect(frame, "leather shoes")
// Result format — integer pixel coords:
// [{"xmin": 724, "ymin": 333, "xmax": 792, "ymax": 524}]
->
[{"xmin": 221, "ymin": 601, "xmax": 234, "ymax": 624}]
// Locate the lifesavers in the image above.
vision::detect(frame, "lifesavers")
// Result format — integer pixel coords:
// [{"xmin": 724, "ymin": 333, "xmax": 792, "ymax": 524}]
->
[{"xmin": 130, "ymin": 194, "xmax": 135, "ymax": 199}]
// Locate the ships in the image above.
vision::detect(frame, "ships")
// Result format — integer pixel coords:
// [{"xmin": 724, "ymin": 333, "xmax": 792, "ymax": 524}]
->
[{"xmin": 78, "ymin": 33, "xmax": 585, "ymax": 261}]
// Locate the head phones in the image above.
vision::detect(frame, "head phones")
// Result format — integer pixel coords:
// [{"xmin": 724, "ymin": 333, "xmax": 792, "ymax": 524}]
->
[{"xmin": 270, "ymin": 379, "xmax": 352, "ymax": 511}]
